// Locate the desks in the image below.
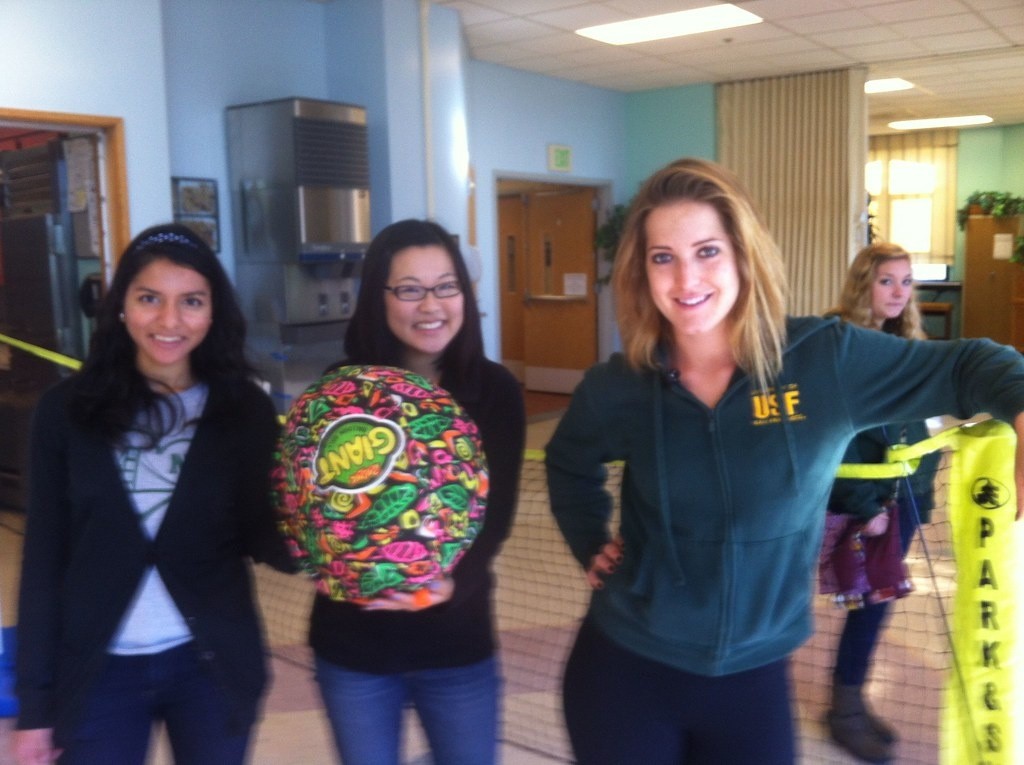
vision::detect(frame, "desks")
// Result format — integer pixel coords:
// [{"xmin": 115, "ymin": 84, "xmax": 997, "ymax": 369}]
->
[{"xmin": 920, "ymin": 301, "xmax": 955, "ymax": 342}]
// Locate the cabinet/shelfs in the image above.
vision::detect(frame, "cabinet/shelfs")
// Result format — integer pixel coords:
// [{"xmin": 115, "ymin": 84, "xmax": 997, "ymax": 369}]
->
[{"xmin": 964, "ymin": 214, "xmax": 1024, "ymax": 354}]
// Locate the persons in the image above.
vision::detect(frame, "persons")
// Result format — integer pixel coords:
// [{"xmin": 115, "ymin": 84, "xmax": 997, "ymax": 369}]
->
[
  {"xmin": 12, "ymin": 223, "xmax": 302, "ymax": 765},
  {"xmin": 544, "ymin": 158, "xmax": 1024, "ymax": 765},
  {"xmin": 307, "ymin": 220, "xmax": 526, "ymax": 764}
]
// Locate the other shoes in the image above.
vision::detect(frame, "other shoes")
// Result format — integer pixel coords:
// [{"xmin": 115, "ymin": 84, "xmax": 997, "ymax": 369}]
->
[
  {"xmin": 874, "ymin": 713, "xmax": 901, "ymax": 744},
  {"xmin": 829, "ymin": 700, "xmax": 894, "ymax": 761}
]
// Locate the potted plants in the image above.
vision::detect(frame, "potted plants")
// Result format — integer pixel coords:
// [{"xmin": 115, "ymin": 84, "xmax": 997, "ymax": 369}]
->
[{"xmin": 957, "ymin": 191, "xmax": 1024, "ymax": 233}]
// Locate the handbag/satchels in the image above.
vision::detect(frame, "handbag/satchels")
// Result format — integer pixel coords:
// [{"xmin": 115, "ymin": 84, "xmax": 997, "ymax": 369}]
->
[{"xmin": 819, "ymin": 504, "xmax": 917, "ymax": 611}]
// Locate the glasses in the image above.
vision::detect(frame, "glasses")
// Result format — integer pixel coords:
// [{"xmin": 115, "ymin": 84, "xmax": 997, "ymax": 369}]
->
[{"xmin": 384, "ymin": 279, "xmax": 464, "ymax": 301}]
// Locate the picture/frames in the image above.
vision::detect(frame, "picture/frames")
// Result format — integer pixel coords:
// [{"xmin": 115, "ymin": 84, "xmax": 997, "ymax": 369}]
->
[{"xmin": 171, "ymin": 176, "xmax": 223, "ymax": 256}]
